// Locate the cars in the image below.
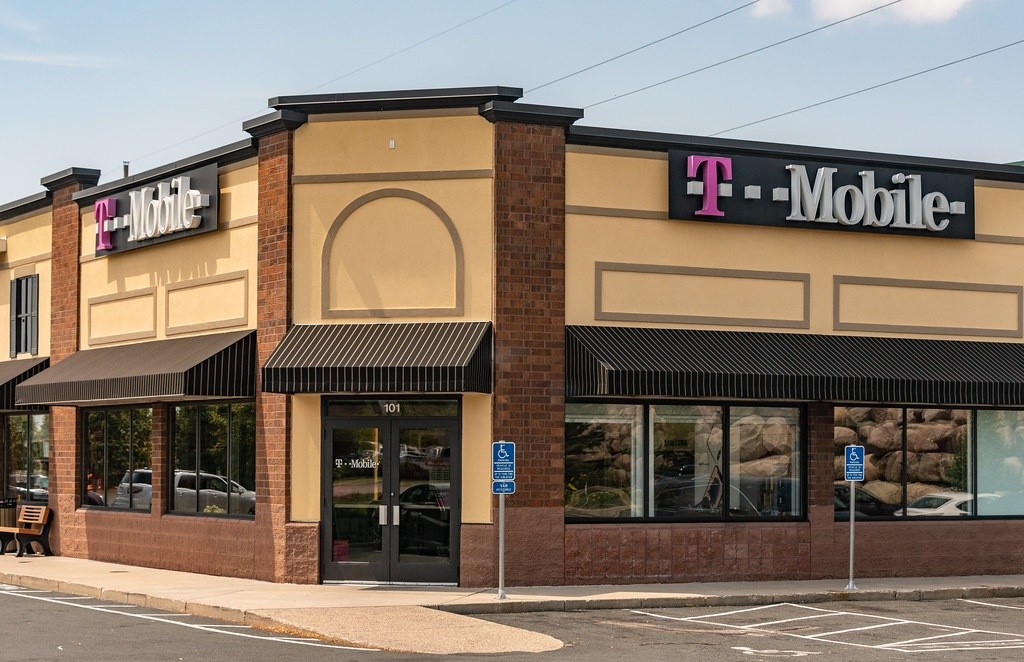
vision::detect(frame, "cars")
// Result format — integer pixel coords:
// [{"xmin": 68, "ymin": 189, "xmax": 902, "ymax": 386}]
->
[
  {"xmin": 356, "ymin": 440, "xmax": 451, "ymax": 463},
  {"xmin": 562, "ymin": 443, "xmax": 905, "ymax": 521},
  {"xmin": 394, "ymin": 481, "xmax": 452, "ymax": 546},
  {"xmin": 6, "ymin": 473, "xmax": 106, "ymax": 508},
  {"xmin": 893, "ymin": 491, "xmax": 1004, "ymax": 522}
]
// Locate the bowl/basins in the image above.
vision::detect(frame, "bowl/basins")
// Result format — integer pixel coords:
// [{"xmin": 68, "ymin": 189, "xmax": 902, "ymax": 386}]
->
[{"xmin": 730, "ymin": 508, "xmax": 740, "ymax": 513}]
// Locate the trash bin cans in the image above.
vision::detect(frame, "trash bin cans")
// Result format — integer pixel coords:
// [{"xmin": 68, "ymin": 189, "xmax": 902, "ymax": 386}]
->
[{"xmin": 0, "ymin": 497, "xmax": 16, "ymax": 552}]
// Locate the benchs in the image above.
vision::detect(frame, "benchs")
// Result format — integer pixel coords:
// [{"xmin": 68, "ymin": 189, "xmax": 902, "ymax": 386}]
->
[{"xmin": 0, "ymin": 505, "xmax": 54, "ymax": 557}]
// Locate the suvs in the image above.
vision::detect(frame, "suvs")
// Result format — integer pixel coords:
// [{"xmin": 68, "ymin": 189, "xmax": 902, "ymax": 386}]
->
[{"xmin": 110, "ymin": 466, "xmax": 256, "ymax": 514}]
